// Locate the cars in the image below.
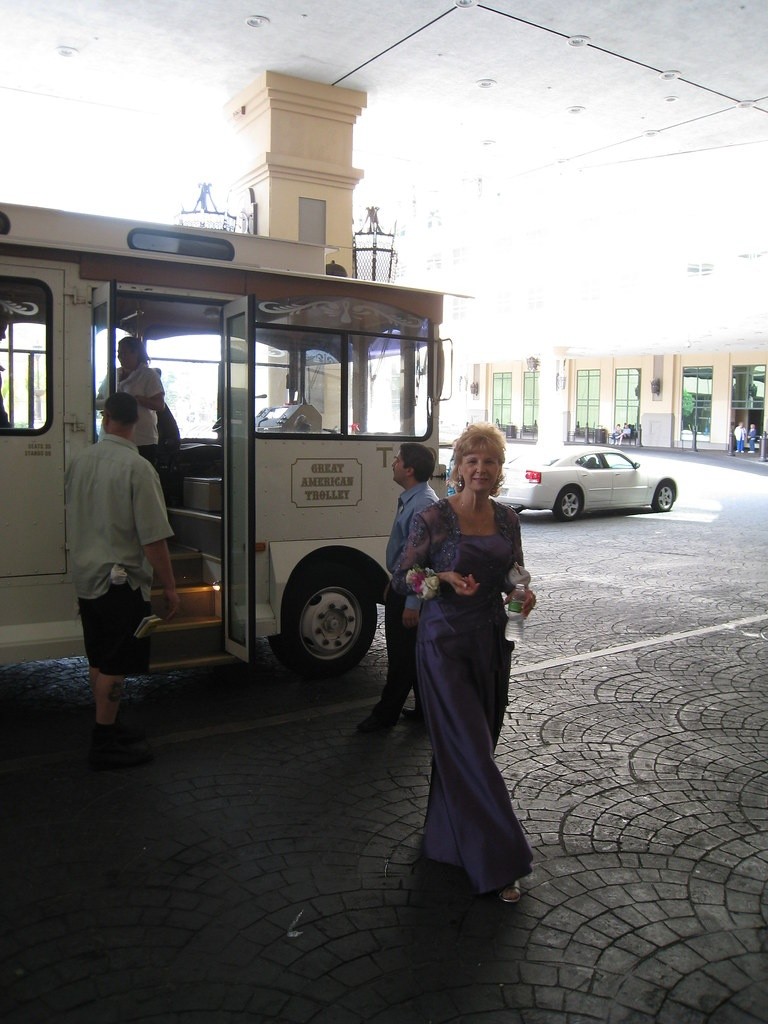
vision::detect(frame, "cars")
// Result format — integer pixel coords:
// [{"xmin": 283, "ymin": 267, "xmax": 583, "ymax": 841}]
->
[{"xmin": 487, "ymin": 444, "xmax": 678, "ymax": 522}]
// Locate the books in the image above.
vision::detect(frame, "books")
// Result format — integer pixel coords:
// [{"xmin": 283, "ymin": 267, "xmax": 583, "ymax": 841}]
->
[{"xmin": 133, "ymin": 614, "xmax": 164, "ymax": 638}]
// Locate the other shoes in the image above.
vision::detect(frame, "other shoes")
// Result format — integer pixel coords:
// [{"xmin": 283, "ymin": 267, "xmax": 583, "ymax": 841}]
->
[
  {"xmin": 735, "ymin": 450, "xmax": 738, "ymax": 452},
  {"xmin": 748, "ymin": 452, "xmax": 754, "ymax": 453},
  {"xmin": 402, "ymin": 708, "xmax": 424, "ymax": 722},
  {"xmin": 741, "ymin": 450, "xmax": 745, "ymax": 453},
  {"xmin": 357, "ymin": 716, "xmax": 395, "ymax": 732},
  {"xmin": 498, "ymin": 880, "xmax": 520, "ymax": 903}
]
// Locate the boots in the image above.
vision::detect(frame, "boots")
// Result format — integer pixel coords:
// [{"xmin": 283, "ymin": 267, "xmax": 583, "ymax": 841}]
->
[{"xmin": 86, "ymin": 709, "xmax": 146, "ymax": 768}]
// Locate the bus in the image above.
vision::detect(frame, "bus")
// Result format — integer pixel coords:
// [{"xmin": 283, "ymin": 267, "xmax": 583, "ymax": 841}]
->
[{"xmin": 0, "ymin": 183, "xmax": 452, "ymax": 681}]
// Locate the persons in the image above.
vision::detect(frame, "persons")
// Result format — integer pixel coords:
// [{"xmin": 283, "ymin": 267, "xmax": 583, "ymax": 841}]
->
[
  {"xmin": 392, "ymin": 422, "xmax": 537, "ymax": 905},
  {"xmin": 62, "ymin": 393, "xmax": 182, "ymax": 767},
  {"xmin": 610, "ymin": 423, "xmax": 631, "ymax": 446},
  {"xmin": 748, "ymin": 424, "xmax": 756, "ymax": 453},
  {"xmin": 733, "ymin": 422, "xmax": 747, "ymax": 453},
  {"xmin": 353, "ymin": 441, "xmax": 441, "ymax": 733},
  {"xmin": 95, "ymin": 336, "xmax": 180, "ymax": 503}
]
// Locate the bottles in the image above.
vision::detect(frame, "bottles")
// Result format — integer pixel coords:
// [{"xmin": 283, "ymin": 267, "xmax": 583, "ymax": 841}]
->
[
  {"xmin": 504, "ymin": 584, "xmax": 525, "ymax": 641},
  {"xmin": 351, "ymin": 424, "xmax": 356, "ymax": 435}
]
